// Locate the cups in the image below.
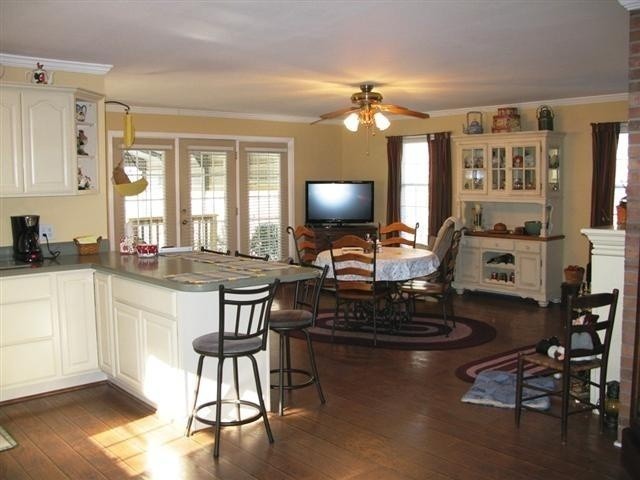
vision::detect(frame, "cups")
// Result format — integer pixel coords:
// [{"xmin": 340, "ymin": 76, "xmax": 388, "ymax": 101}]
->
[{"xmin": 462, "ymin": 111, "xmax": 483, "ymax": 134}]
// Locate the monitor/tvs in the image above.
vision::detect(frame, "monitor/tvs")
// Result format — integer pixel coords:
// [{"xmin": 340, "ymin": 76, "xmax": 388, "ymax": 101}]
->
[{"xmin": 304, "ymin": 180, "xmax": 374, "ymax": 228}]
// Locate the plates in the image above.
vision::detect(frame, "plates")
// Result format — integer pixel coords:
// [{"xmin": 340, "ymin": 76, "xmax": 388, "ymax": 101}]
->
[{"xmin": 487, "ymin": 229, "xmax": 512, "ymax": 234}]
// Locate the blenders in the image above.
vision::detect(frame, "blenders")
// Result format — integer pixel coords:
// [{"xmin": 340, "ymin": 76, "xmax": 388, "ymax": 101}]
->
[{"xmin": 471, "ymin": 204, "xmax": 484, "ymax": 233}]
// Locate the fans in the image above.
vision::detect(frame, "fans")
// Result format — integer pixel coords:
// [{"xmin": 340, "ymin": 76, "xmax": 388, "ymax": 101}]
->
[{"xmin": 312, "ymin": 84, "xmax": 431, "ymax": 126}]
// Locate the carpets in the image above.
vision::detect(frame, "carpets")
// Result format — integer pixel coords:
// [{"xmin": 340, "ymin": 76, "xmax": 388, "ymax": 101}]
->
[
  {"xmin": 456, "ymin": 341, "xmax": 566, "ymax": 395},
  {"xmin": 280, "ymin": 310, "xmax": 495, "ymax": 351}
]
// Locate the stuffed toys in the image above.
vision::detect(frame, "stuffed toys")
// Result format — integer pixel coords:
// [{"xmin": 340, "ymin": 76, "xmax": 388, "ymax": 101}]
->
[{"xmin": 535, "ymin": 311, "xmax": 606, "ymax": 365}]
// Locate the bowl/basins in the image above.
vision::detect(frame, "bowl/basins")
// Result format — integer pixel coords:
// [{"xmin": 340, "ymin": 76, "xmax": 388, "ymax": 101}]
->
[
  {"xmin": 135, "ymin": 243, "xmax": 159, "ymax": 258},
  {"xmin": 524, "ymin": 220, "xmax": 541, "ymax": 234}
]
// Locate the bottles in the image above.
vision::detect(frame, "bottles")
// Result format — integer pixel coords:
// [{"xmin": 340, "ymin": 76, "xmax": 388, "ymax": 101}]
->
[{"xmin": 603, "ymin": 381, "xmax": 620, "ymax": 436}]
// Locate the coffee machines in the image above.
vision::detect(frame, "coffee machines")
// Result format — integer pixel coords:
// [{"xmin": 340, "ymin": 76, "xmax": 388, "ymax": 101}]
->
[{"xmin": 10, "ymin": 214, "xmax": 43, "ymax": 263}]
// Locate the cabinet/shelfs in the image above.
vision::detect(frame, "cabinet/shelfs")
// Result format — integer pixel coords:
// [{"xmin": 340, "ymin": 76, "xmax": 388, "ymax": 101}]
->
[
  {"xmin": 0, "ymin": 272, "xmax": 60, "ymax": 410},
  {"xmin": 56, "ymin": 269, "xmax": 113, "ymax": 399},
  {"xmin": 449, "ymin": 130, "xmax": 564, "ymax": 312},
  {"xmin": 0, "ymin": 82, "xmax": 104, "ymax": 200},
  {"xmin": 114, "ymin": 277, "xmax": 272, "ymax": 436}
]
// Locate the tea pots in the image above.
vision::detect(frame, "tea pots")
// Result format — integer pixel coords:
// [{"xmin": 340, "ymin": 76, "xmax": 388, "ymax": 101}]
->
[{"xmin": 23, "ymin": 62, "xmax": 55, "ymax": 86}]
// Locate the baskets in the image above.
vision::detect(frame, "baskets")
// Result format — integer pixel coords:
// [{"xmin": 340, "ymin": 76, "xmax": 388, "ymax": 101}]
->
[{"xmin": 73, "ymin": 236, "xmax": 102, "ymax": 256}]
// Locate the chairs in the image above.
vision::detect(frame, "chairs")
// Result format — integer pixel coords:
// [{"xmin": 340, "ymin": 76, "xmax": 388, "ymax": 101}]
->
[
  {"xmin": 284, "ymin": 217, "xmax": 459, "ymax": 337},
  {"xmin": 505, "ymin": 290, "xmax": 617, "ymax": 446},
  {"xmin": 181, "ymin": 246, "xmax": 328, "ymax": 459}
]
